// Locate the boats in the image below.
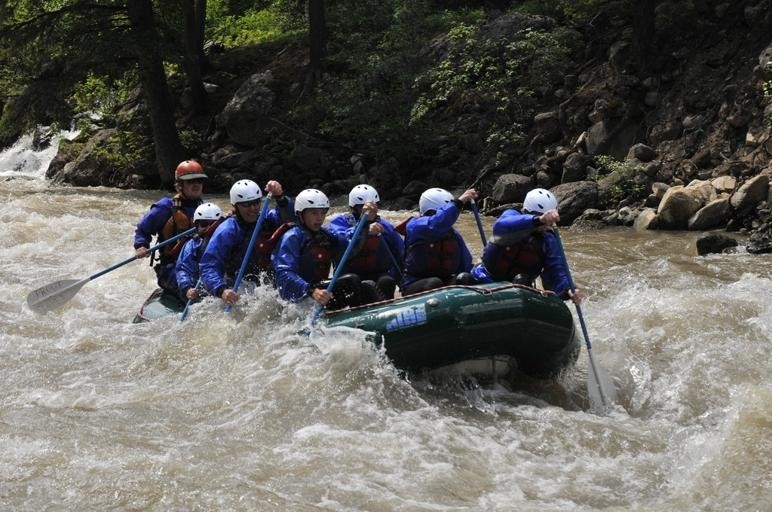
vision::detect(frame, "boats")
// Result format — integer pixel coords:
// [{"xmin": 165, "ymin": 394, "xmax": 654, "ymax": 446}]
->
[{"xmin": 133, "ymin": 280, "xmax": 582, "ymax": 386}]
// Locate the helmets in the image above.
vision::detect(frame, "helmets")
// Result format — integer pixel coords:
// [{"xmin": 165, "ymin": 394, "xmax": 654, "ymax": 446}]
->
[
  {"xmin": 193, "ymin": 202, "xmax": 222, "ymax": 222},
  {"xmin": 294, "ymin": 188, "xmax": 330, "ymax": 216},
  {"xmin": 230, "ymin": 179, "xmax": 262, "ymax": 205},
  {"xmin": 420, "ymin": 187, "xmax": 454, "ymax": 216},
  {"xmin": 523, "ymin": 188, "xmax": 556, "ymax": 214},
  {"xmin": 349, "ymin": 183, "xmax": 380, "ymax": 207},
  {"xmin": 176, "ymin": 161, "xmax": 208, "ymax": 179}
]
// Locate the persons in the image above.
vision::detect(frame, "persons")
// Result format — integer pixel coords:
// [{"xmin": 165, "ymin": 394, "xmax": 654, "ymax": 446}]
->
[
  {"xmin": 400, "ymin": 188, "xmax": 478, "ymax": 297},
  {"xmin": 133, "ymin": 160, "xmax": 296, "ymax": 314},
  {"xmin": 275, "ymin": 182, "xmax": 404, "ymax": 309},
  {"xmin": 471, "ymin": 187, "xmax": 584, "ymax": 306}
]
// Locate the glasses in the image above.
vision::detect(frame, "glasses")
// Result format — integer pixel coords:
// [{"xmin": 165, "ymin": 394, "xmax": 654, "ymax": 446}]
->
[
  {"xmin": 185, "ymin": 179, "xmax": 203, "ymax": 184},
  {"xmin": 198, "ymin": 220, "xmax": 214, "ymax": 228}
]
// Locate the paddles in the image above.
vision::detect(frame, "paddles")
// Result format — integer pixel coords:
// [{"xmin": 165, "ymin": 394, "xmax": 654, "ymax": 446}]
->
[
  {"xmin": 550, "ymin": 222, "xmax": 618, "ymax": 407},
  {"xmin": 29, "ymin": 226, "xmax": 196, "ymax": 316}
]
